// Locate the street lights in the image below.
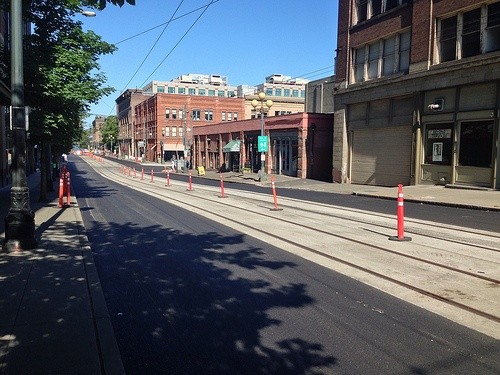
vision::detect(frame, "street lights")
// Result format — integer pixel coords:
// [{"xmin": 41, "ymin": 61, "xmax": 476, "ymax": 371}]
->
[
  {"xmin": 251, "ymin": 92, "xmax": 273, "ymax": 182},
  {"xmin": 109, "ymin": 135, "xmax": 114, "ymax": 155}
]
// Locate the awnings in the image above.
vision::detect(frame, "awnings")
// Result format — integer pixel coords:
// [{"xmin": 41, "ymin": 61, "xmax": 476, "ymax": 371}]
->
[{"xmin": 223, "ymin": 139, "xmax": 240, "ymax": 152}]
